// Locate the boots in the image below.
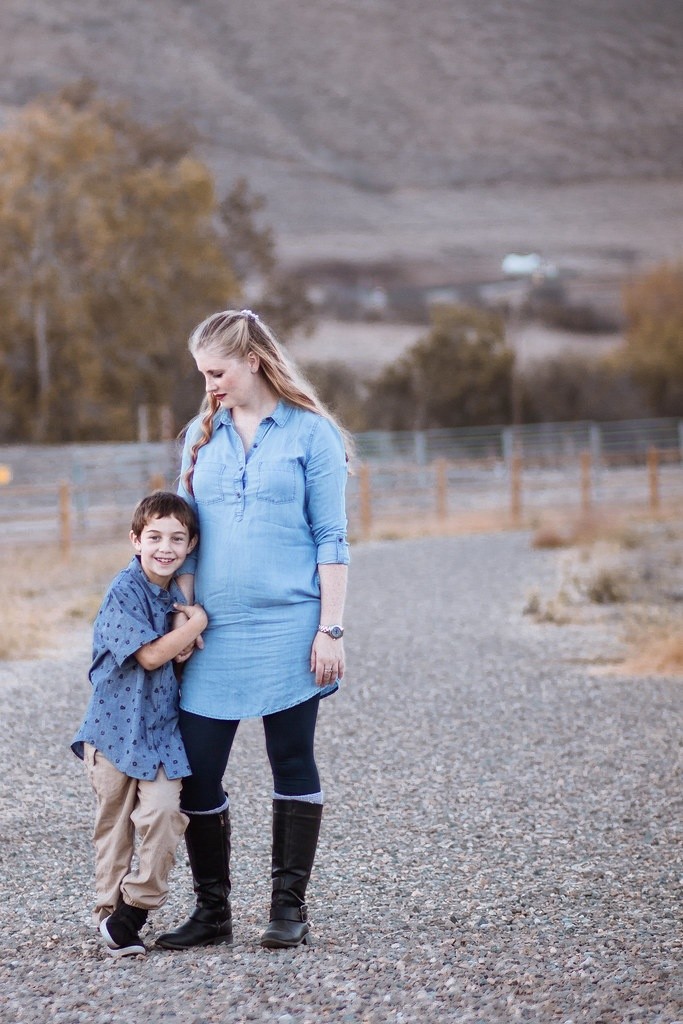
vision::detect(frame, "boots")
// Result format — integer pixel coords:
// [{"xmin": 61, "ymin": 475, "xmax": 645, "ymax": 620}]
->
[
  {"xmin": 155, "ymin": 791, "xmax": 232, "ymax": 948},
  {"xmin": 260, "ymin": 791, "xmax": 323, "ymax": 945}
]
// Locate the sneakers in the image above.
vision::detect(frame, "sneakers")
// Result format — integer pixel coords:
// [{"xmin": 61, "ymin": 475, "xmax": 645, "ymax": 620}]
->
[
  {"xmin": 106, "ymin": 934, "xmax": 147, "ymax": 956},
  {"xmin": 100, "ymin": 900, "xmax": 149, "ymax": 949}
]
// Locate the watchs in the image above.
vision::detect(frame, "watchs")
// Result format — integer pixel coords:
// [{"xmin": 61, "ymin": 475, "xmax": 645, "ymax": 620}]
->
[{"xmin": 318, "ymin": 624, "xmax": 345, "ymax": 639}]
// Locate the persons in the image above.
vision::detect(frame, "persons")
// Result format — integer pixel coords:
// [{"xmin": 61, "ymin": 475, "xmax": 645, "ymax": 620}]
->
[
  {"xmin": 70, "ymin": 491, "xmax": 209, "ymax": 958},
  {"xmin": 154, "ymin": 309, "xmax": 352, "ymax": 948}
]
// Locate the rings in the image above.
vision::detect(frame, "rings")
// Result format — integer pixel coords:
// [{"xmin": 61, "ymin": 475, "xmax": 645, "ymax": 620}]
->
[{"xmin": 325, "ymin": 670, "xmax": 333, "ymax": 672}]
